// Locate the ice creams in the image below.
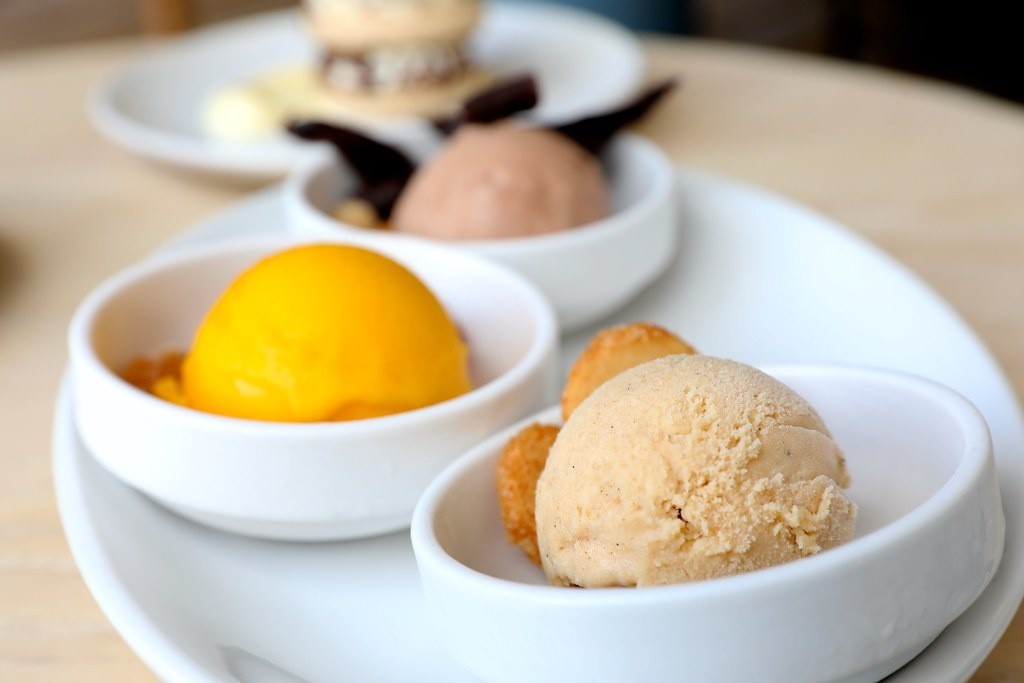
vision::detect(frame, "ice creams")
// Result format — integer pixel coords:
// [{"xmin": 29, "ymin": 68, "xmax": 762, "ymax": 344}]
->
[{"xmin": 123, "ymin": 2, "xmax": 855, "ymax": 590}]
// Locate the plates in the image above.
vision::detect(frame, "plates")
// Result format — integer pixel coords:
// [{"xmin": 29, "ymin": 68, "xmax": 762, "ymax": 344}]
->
[
  {"xmin": 52, "ymin": 159, "xmax": 1024, "ymax": 682},
  {"xmin": 83, "ymin": 0, "xmax": 647, "ymax": 183}
]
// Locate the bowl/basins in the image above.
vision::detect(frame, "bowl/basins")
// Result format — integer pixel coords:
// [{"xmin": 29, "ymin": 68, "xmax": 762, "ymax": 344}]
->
[
  {"xmin": 65, "ymin": 231, "xmax": 558, "ymax": 542},
  {"xmin": 284, "ymin": 139, "xmax": 676, "ymax": 328},
  {"xmin": 410, "ymin": 363, "xmax": 1003, "ymax": 683}
]
